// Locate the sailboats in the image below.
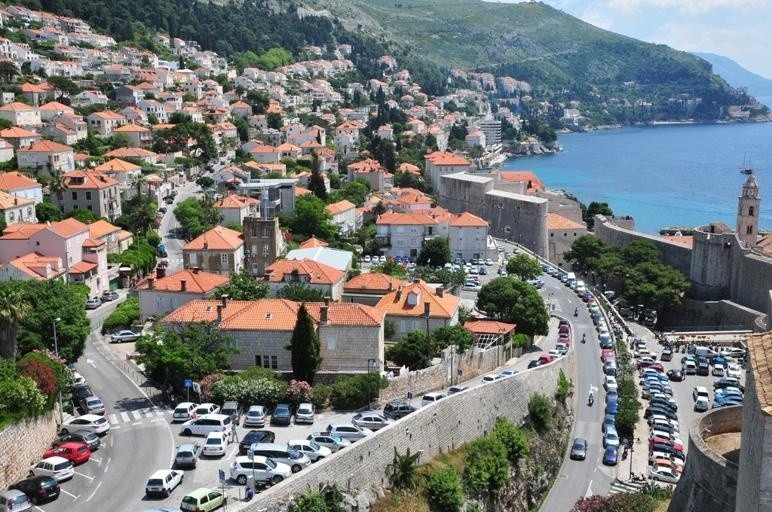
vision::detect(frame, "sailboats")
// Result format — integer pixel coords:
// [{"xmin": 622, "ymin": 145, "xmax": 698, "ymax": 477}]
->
[{"xmin": 740, "ymin": 151, "xmax": 753, "ymax": 176}]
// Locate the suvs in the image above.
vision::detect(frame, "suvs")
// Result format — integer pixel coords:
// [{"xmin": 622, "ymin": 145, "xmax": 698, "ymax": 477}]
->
[
  {"xmin": 51, "ymin": 430, "xmax": 100, "ymax": 452},
  {"xmin": 146, "ymin": 469, "xmax": 184, "ymax": 498},
  {"xmin": 0, "ymin": 489, "xmax": 32, "ymax": 512},
  {"xmin": 59, "ymin": 413, "xmax": 109, "ymax": 438},
  {"xmin": 8, "ymin": 475, "xmax": 60, "ymax": 506},
  {"xmin": 383, "ymin": 402, "xmax": 418, "ymax": 420},
  {"xmin": 179, "ymin": 488, "xmax": 229, "ymax": 512},
  {"xmin": 174, "ymin": 444, "xmax": 202, "ymax": 470},
  {"xmin": 44, "ymin": 441, "xmax": 90, "ymax": 467},
  {"xmin": 327, "ymin": 423, "xmax": 374, "ymax": 443},
  {"xmin": 229, "ymin": 455, "xmax": 292, "ymax": 487},
  {"xmin": 28, "ymin": 456, "xmax": 74, "ymax": 483},
  {"xmin": 247, "ymin": 442, "xmax": 311, "ymax": 472}
]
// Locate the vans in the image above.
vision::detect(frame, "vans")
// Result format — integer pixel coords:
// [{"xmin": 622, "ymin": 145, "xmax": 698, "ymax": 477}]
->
[
  {"xmin": 448, "ymin": 385, "xmax": 468, "ymax": 398},
  {"xmin": 102, "ymin": 291, "xmax": 118, "ymax": 302},
  {"xmin": 181, "ymin": 414, "xmax": 232, "ymax": 438},
  {"xmin": 422, "ymin": 391, "xmax": 445, "ymax": 408}
]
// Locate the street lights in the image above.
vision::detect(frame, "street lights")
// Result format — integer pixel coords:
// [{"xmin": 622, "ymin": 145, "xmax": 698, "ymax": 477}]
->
[
  {"xmin": 65, "ymin": 250, "xmax": 70, "ymax": 288},
  {"xmin": 367, "ymin": 358, "xmax": 377, "ymax": 412},
  {"xmin": 419, "ymin": 312, "xmax": 433, "ymax": 366},
  {"xmin": 136, "ymin": 230, "xmax": 141, "ymax": 280},
  {"xmin": 450, "ymin": 344, "xmax": 460, "ymax": 387},
  {"xmin": 52, "ymin": 317, "xmax": 63, "ymax": 424}
]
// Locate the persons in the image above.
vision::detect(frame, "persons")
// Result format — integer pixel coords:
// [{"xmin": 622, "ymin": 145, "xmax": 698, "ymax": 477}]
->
[
  {"xmin": 288, "ymin": 412, "xmax": 295, "ymax": 429},
  {"xmin": 191, "ymin": 411, "xmax": 196, "ymax": 419},
  {"xmin": 582, "ymin": 332, "xmax": 586, "ymax": 341},
  {"xmin": 575, "ymin": 307, "xmax": 579, "ymax": 317},
  {"xmin": 681, "ymin": 368, "xmax": 688, "ymax": 380},
  {"xmin": 230, "ymin": 411, "xmax": 237, "ymax": 424},
  {"xmin": 230, "ymin": 422, "xmax": 240, "ymax": 443},
  {"xmin": 588, "ymin": 393, "xmax": 594, "ymax": 404}
]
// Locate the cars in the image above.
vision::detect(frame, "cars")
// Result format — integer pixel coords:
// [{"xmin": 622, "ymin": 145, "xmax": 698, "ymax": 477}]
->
[
  {"xmin": 356, "ymin": 253, "xmax": 482, "ymax": 293},
  {"xmin": 239, "ymin": 430, "xmax": 275, "ymax": 454},
  {"xmin": 307, "ymin": 432, "xmax": 352, "ymax": 453},
  {"xmin": 202, "ymin": 432, "xmax": 229, "ymax": 458},
  {"xmin": 72, "ymin": 383, "xmax": 93, "ymax": 406},
  {"xmin": 287, "ymin": 440, "xmax": 331, "ymax": 462},
  {"xmin": 171, "ymin": 400, "xmax": 316, "ymax": 428},
  {"xmin": 86, "ymin": 299, "xmax": 102, "ymax": 309},
  {"xmin": 352, "ymin": 412, "xmax": 395, "ymax": 431},
  {"xmin": 154, "ymin": 155, "xmax": 235, "ymax": 269},
  {"xmin": 111, "ymin": 329, "xmax": 140, "ymax": 344},
  {"xmin": 141, "ymin": 505, "xmax": 182, "ymax": 512},
  {"xmin": 80, "ymin": 396, "xmax": 105, "ymax": 415}
]
